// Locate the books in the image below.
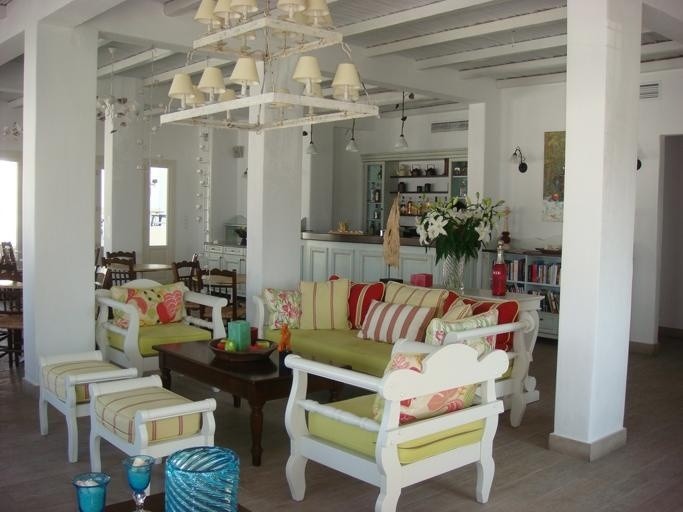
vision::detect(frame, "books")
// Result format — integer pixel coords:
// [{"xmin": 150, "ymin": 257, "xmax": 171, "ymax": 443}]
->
[
  {"xmin": 504, "ymin": 258, "xmax": 525, "ymax": 282},
  {"xmin": 529, "ymin": 290, "xmax": 560, "ymax": 313},
  {"xmin": 528, "ymin": 263, "xmax": 562, "ymax": 283},
  {"xmin": 505, "ymin": 285, "xmax": 525, "ymax": 294}
]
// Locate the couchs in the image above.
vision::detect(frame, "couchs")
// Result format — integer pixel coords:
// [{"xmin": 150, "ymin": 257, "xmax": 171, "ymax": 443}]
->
[
  {"xmin": 253, "ymin": 282, "xmax": 540, "ymax": 427},
  {"xmin": 284, "ymin": 343, "xmax": 509, "ymax": 512}
]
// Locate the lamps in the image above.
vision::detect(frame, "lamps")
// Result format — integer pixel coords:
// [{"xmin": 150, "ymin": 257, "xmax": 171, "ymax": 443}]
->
[
  {"xmin": 345, "ymin": 119, "xmax": 361, "ymax": 152},
  {"xmin": 160, "ymin": 0, "xmax": 380, "ymax": 132},
  {"xmin": 96, "ymin": 46, "xmax": 138, "ymax": 138},
  {"xmin": 135, "ymin": 46, "xmax": 168, "ymax": 187},
  {"xmin": 507, "ymin": 146, "xmax": 528, "ymax": 173},
  {"xmin": 394, "ymin": 91, "xmax": 414, "ymax": 149},
  {"xmin": 4, "ymin": 62, "xmax": 25, "ymax": 143},
  {"xmin": 302, "ymin": 124, "xmax": 319, "ymax": 155}
]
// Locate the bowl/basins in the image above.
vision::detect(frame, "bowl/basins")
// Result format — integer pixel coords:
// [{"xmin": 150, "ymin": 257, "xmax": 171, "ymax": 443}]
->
[{"xmin": 208, "ymin": 337, "xmax": 278, "ymax": 362}]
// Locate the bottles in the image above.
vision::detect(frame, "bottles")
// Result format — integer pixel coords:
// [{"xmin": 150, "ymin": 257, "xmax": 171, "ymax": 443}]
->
[
  {"xmin": 369, "ymin": 183, "xmax": 375, "ymax": 202},
  {"xmin": 377, "ymin": 164, "xmax": 382, "ymax": 182},
  {"xmin": 373, "ymin": 183, "xmax": 381, "ymax": 202},
  {"xmin": 491, "ymin": 241, "xmax": 508, "ymax": 296},
  {"xmin": 373, "ymin": 204, "xmax": 379, "ymax": 220},
  {"xmin": 406, "ymin": 197, "xmax": 412, "ymax": 215},
  {"xmin": 369, "ymin": 221, "xmax": 374, "ymax": 236},
  {"xmin": 425, "ymin": 198, "xmax": 430, "ymax": 214},
  {"xmin": 400, "ymin": 196, "xmax": 406, "ymax": 215},
  {"xmin": 416, "ymin": 196, "xmax": 423, "ymax": 217},
  {"xmin": 443, "ymin": 196, "xmax": 449, "ymax": 208},
  {"xmin": 434, "ymin": 197, "xmax": 439, "ymax": 208}
]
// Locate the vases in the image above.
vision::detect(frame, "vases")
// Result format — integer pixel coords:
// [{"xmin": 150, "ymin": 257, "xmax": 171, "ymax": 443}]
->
[{"xmin": 442, "ymin": 246, "xmax": 465, "ymax": 296}]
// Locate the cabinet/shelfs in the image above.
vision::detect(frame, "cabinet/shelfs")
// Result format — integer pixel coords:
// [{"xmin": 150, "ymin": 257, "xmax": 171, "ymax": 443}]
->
[
  {"xmin": 204, "ymin": 241, "xmax": 246, "ymax": 297},
  {"xmin": 481, "ymin": 244, "xmax": 561, "ymax": 341},
  {"xmin": 361, "ymin": 150, "xmax": 468, "ymax": 236}
]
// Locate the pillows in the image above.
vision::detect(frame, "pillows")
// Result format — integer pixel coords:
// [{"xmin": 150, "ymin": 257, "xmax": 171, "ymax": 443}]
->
[
  {"xmin": 301, "ymin": 280, "xmax": 352, "ymax": 330},
  {"xmin": 425, "ymin": 309, "xmax": 499, "ymax": 353},
  {"xmin": 384, "ymin": 280, "xmax": 449, "ymax": 319},
  {"xmin": 357, "ymin": 297, "xmax": 435, "ymax": 342},
  {"xmin": 111, "ymin": 282, "xmax": 188, "ymax": 328},
  {"xmin": 371, "ymin": 337, "xmax": 492, "ymax": 427},
  {"xmin": 263, "ymin": 287, "xmax": 300, "ymax": 329},
  {"xmin": 329, "ymin": 274, "xmax": 385, "ymax": 328},
  {"xmin": 442, "ymin": 290, "xmax": 520, "ymax": 352}
]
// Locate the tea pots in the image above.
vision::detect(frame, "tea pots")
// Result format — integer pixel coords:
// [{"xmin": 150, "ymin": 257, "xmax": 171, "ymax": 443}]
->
[
  {"xmin": 409, "ymin": 164, "xmax": 422, "ymax": 177},
  {"xmin": 395, "ymin": 164, "xmax": 407, "ymax": 176},
  {"xmin": 424, "ymin": 163, "xmax": 436, "ymax": 176}
]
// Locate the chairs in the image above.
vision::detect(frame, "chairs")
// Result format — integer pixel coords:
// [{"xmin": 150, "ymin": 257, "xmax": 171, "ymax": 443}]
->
[
  {"xmin": 0, "ymin": 288, "xmax": 23, "ymax": 367},
  {"xmin": 106, "ymin": 251, "xmax": 137, "ymax": 280},
  {"xmin": 172, "ymin": 260, "xmax": 203, "ymax": 316},
  {"xmin": 101, "ymin": 257, "xmax": 134, "ymax": 319},
  {"xmin": 93, "ymin": 279, "xmax": 228, "ymax": 377},
  {"xmin": 196, "ymin": 269, "xmax": 246, "ymax": 338},
  {"xmin": 0, "ymin": 241, "xmax": 22, "ymax": 281},
  {"xmin": 0, "ymin": 262, "xmax": 17, "ymax": 312},
  {"xmin": 95, "ymin": 265, "xmax": 110, "ymax": 319}
]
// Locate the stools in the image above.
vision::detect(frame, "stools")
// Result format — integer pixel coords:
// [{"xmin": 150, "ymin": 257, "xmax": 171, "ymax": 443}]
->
[
  {"xmin": 38, "ymin": 349, "xmax": 138, "ymax": 462},
  {"xmin": 89, "ymin": 374, "xmax": 217, "ymax": 497}
]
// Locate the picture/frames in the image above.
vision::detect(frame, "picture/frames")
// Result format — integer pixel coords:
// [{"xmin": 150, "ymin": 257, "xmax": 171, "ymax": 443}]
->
[{"xmin": 541, "ymin": 131, "xmax": 566, "ymax": 221}]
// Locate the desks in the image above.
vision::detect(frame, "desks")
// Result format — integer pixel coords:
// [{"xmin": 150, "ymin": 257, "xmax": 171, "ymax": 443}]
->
[
  {"xmin": 97, "ymin": 262, "xmax": 172, "ymax": 272},
  {"xmin": 406, "ymin": 283, "xmax": 545, "ymax": 428}
]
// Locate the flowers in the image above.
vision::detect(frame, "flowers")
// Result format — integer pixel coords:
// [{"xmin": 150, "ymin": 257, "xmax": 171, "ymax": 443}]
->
[{"xmin": 412, "ymin": 191, "xmax": 510, "ymax": 265}]
